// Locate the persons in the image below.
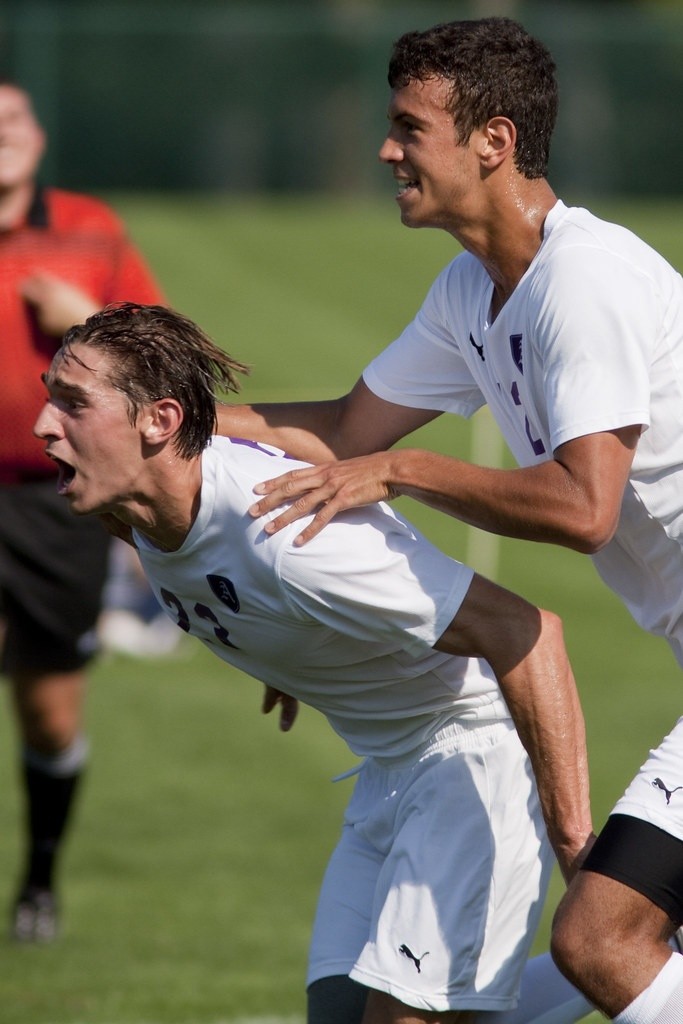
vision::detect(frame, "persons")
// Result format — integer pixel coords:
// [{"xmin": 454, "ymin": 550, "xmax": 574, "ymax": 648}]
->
[
  {"xmin": 209, "ymin": 16, "xmax": 682, "ymax": 1024},
  {"xmin": 34, "ymin": 303, "xmax": 683, "ymax": 1024},
  {"xmin": 0, "ymin": 80, "xmax": 169, "ymax": 944}
]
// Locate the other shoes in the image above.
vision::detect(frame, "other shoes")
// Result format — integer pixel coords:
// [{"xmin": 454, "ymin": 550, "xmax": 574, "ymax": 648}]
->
[{"xmin": 12, "ymin": 888, "xmax": 53, "ymax": 942}]
